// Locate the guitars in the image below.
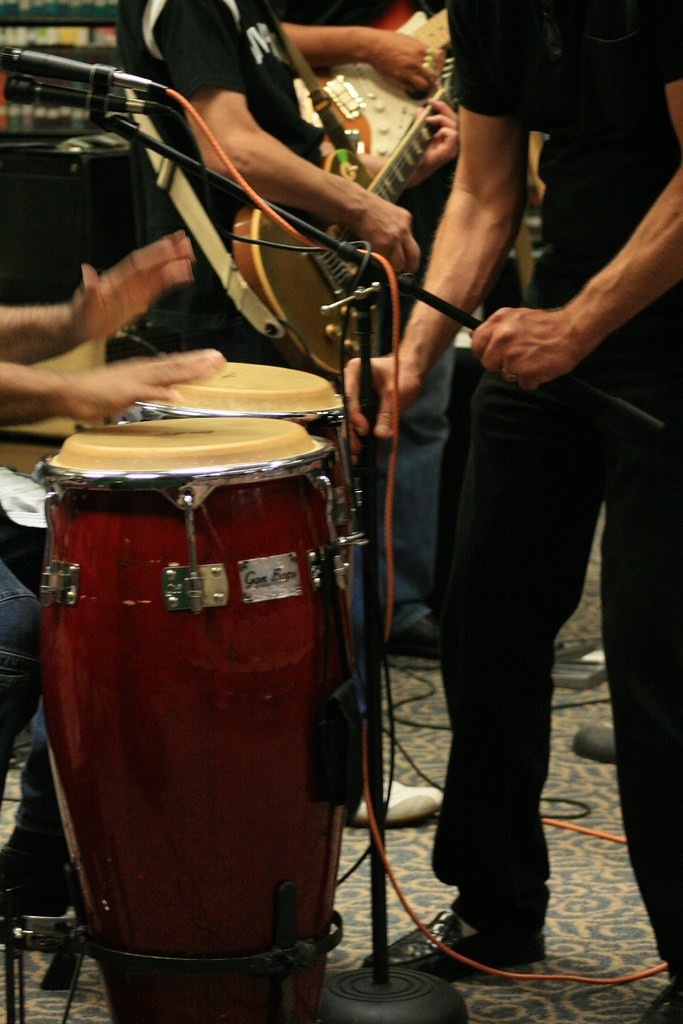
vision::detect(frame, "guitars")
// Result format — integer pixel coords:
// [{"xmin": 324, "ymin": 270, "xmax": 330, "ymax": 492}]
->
[{"xmin": 232, "ymin": 46, "xmax": 457, "ymax": 380}]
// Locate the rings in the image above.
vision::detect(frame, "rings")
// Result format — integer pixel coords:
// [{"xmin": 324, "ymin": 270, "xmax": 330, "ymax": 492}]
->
[{"xmin": 501, "ymin": 367, "xmax": 517, "ymax": 383}]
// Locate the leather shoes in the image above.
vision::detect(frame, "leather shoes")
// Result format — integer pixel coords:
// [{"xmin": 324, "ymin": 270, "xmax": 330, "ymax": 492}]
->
[
  {"xmin": 639, "ymin": 984, "xmax": 683, "ymax": 1024},
  {"xmin": 362, "ymin": 910, "xmax": 546, "ymax": 982}
]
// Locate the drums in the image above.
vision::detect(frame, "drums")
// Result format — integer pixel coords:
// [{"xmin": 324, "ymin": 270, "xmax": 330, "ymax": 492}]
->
[
  {"xmin": 122, "ymin": 361, "xmax": 355, "ymax": 605},
  {"xmin": 32, "ymin": 416, "xmax": 353, "ymax": 1023}
]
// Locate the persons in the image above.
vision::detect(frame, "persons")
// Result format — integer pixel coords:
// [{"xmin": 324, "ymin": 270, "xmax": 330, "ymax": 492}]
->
[
  {"xmin": 345, "ymin": 1, "xmax": 683, "ymax": 1023},
  {"xmin": 1, "ymin": 0, "xmax": 683, "ymax": 939}
]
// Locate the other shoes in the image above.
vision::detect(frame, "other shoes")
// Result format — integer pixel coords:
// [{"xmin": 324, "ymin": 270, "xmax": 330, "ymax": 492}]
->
[
  {"xmin": 351, "ymin": 777, "xmax": 445, "ymax": 825},
  {"xmin": 573, "ymin": 726, "xmax": 617, "ymax": 763},
  {"xmin": 0, "ymin": 846, "xmax": 71, "ymax": 916},
  {"xmin": 388, "ymin": 618, "xmax": 444, "ymax": 669}
]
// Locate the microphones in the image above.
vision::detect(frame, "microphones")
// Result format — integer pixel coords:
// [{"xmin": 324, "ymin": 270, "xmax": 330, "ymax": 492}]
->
[
  {"xmin": 2, "ymin": 48, "xmax": 169, "ymax": 95},
  {"xmin": 4, "ymin": 72, "xmax": 174, "ymax": 115}
]
